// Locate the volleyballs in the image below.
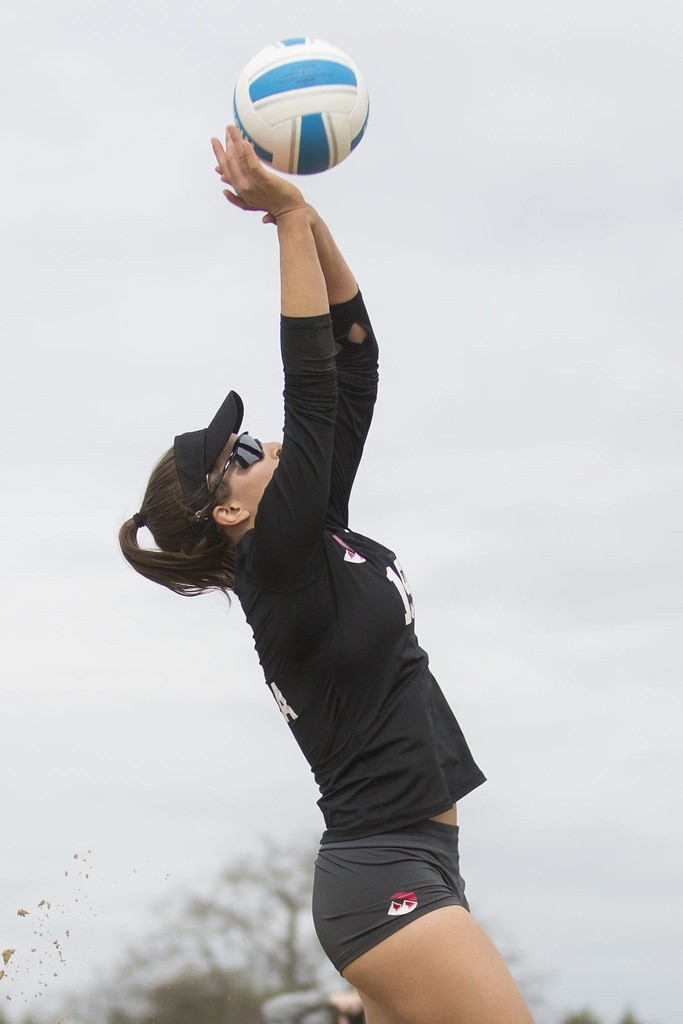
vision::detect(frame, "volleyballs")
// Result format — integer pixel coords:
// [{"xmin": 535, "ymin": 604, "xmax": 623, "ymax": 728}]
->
[{"xmin": 233, "ymin": 37, "xmax": 370, "ymax": 174}]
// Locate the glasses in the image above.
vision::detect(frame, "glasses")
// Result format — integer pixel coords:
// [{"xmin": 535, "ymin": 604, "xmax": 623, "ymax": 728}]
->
[{"xmin": 209, "ymin": 432, "xmax": 264, "ymax": 505}]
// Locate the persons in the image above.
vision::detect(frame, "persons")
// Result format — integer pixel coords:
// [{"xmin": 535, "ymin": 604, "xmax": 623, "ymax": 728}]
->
[{"xmin": 116, "ymin": 125, "xmax": 535, "ymax": 1024}]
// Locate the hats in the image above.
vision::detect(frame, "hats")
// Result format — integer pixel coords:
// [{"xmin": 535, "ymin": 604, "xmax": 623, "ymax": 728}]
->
[{"xmin": 175, "ymin": 391, "xmax": 244, "ymax": 551}]
